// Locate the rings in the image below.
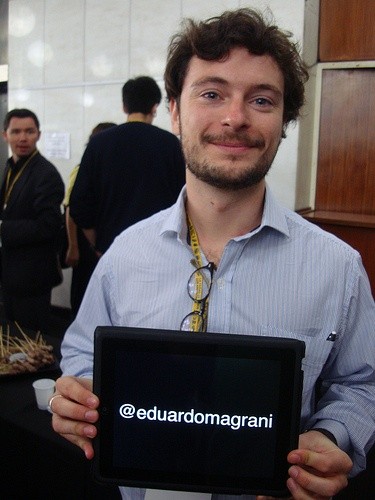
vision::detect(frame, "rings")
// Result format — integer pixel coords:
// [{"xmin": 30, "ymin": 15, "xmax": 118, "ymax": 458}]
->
[{"xmin": 47, "ymin": 394, "xmax": 63, "ymax": 417}]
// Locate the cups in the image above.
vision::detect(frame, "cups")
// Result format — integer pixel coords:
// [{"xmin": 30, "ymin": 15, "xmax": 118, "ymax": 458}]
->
[{"xmin": 32, "ymin": 378, "xmax": 56, "ymax": 412}]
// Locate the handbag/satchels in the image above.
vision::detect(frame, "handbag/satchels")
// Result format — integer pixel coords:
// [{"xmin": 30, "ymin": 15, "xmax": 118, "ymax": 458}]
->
[{"xmin": 60, "ymin": 205, "xmax": 72, "ymax": 269}]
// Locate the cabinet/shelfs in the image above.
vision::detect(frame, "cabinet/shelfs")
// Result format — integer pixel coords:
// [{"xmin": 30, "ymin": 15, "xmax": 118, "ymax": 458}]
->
[{"xmin": 296, "ymin": 0, "xmax": 375, "ymax": 301}]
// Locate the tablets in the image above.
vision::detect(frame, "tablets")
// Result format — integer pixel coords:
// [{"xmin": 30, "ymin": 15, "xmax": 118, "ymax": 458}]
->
[{"xmin": 91, "ymin": 326, "xmax": 305, "ymax": 499}]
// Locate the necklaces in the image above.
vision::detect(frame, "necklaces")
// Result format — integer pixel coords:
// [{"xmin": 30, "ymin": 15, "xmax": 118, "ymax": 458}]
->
[{"xmin": 2, "ymin": 150, "xmax": 37, "ymax": 210}]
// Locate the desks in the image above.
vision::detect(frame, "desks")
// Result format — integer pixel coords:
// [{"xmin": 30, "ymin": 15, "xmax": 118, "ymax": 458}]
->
[{"xmin": 0, "ymin": 302, "xmax": 125, "ymax": 500}]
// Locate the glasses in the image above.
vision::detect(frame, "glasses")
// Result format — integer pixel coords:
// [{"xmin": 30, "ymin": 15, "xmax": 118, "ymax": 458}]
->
[{"xmin": 180, "ymin": 262, "xmax": 217, "ymax": 333}]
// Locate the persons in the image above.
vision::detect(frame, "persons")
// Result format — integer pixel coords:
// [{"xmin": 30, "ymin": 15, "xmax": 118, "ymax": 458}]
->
[
  {"xmin": 61, "ymin": 77, "xmax": 185, "ymax": 319},
  {"xmin": 47, "ymin": 7, "xmax": 374, "ymax": 500},
  {"xmin": 1, "ymin": 108, "xmax": 64, "ymax": 341},
  {"xmin": 62, "ymin": 165, "xmax": 81, "ymax": 333}
]
